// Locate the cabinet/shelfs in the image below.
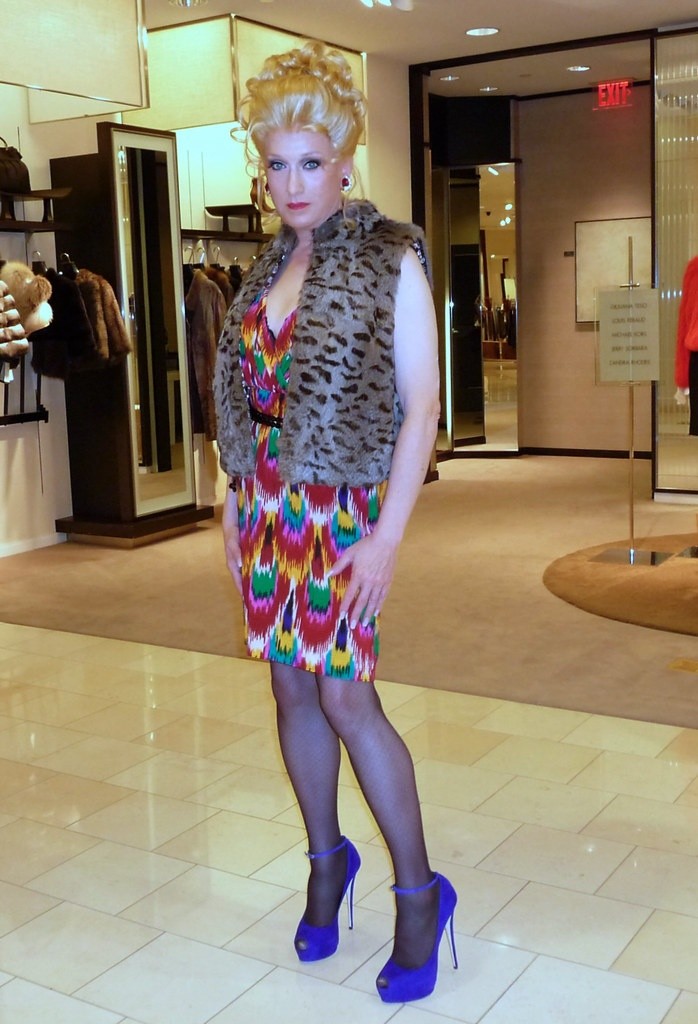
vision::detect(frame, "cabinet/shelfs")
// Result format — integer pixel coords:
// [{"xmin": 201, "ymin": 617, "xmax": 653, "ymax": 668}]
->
[
  {"xmin": 44, "ymin": 122, "xmax": 282, "ymax": 551},
  {"xmin": 1, "ymin": 181, "xmax": 74, "ymax": 430}
]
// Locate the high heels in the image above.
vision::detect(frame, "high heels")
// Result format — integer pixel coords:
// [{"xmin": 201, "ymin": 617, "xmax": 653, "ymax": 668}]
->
[
  {"xmin": 375, "ymin": 873, "xmax": 459, "ymax": 1004},
  {"xmin": 295, "ymin": 837, "xmax": 360, "ymax": 961}
]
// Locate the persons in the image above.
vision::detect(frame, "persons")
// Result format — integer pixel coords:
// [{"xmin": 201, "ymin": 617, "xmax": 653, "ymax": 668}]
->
[
  {"xmin": 209, "ymin": 40, "xmax": 459, "ymax": 1004},
  {"xmin": 674, "ymin": 254, "xmax": 698, "ymax": 436},
  {"xmin": 507, "ymin": 299, "xmax": 517, "ymax": 347}
]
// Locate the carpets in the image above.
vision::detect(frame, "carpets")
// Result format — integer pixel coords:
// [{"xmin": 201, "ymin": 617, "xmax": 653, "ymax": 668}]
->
[{"xmin": 541, "ymin": 532, "xmax": 698, "ymax": 640}]
